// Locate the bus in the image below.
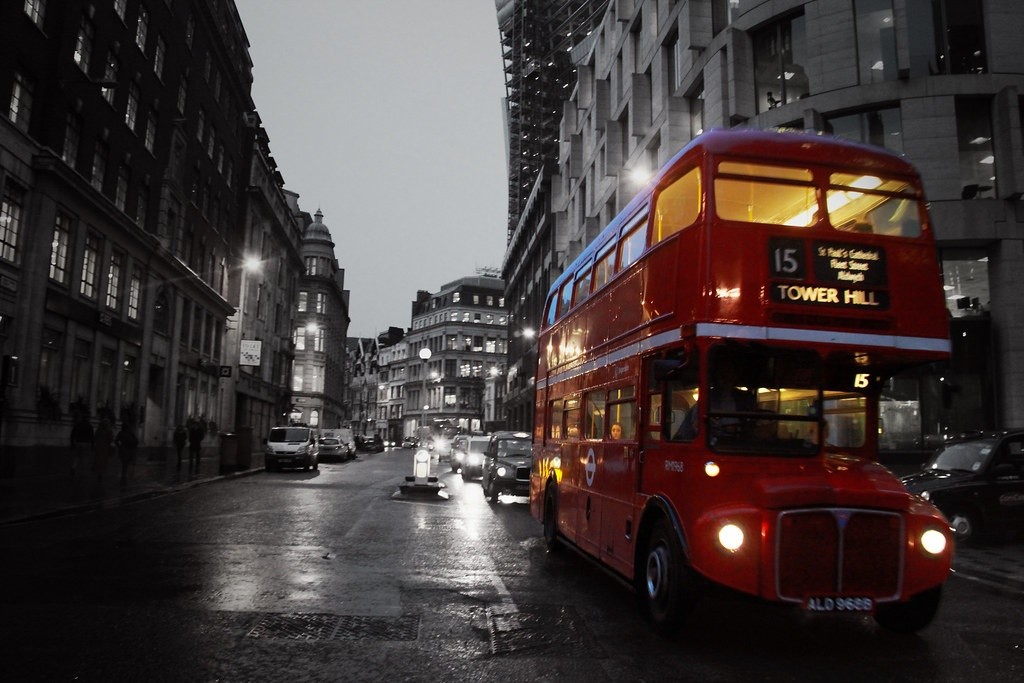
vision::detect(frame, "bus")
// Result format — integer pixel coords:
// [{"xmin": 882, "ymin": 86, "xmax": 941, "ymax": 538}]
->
[{"xmin": 529, "ymin": 126, "xmax": 956, "ymax": 638}]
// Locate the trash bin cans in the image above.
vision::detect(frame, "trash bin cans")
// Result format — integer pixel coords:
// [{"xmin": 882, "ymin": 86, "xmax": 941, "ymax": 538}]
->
[{"xmin": 218, "ymin": 433, "xmax": 239, "ymax": 474}]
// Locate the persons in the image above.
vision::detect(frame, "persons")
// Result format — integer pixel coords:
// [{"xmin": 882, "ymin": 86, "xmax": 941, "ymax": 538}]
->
[
  {"xmin": 188, "ymin": 421, "xmax": 204, "ymax": 475},
  {"xmin": 173, "ymin": 424, "xmax": 186, "ymax": 465},
  {"xmin": 670, "ymin": 369, "xmax": 755, "ymax": 443},
  {"xmin": 95, "ymin": 417, "xmax": 113, "ymax": 483},
  {"xmin": 114, "ymin": 423, "xmax": 138, "ymax": 492},
  {"xmin": 852, "ymin": 222, "xmax": 873, "ymax": 234},
  {"xmin": 70, "ymin": 412, "xmax": 94, "ymax": 476},
  {"xmin": 610, "ymin": 422, "xmax": 625, "ymax": 440}
]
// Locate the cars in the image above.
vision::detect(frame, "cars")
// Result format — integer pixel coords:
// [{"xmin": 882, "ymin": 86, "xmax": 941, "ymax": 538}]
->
[
  {"xmin": 403, "ymin": 437, "xmax": 419, "ymax": 449},
  {"xmin": 318, "ymin": 437, "xmax": 349, "ymax": 463},
  {"xmin": 483, "ymin": 430, "xmax": 530, "ymax": 504},
  {"xmin": 896, "ymin": 427, "xmax": 1024, "ymax": 545},
  {"xmin": 355, "ymin": 434, "xmax": 396, "ymax": 453},
  {"xmin": 450, "ymin": 434, "xmax": 492, "ymax": 481}
]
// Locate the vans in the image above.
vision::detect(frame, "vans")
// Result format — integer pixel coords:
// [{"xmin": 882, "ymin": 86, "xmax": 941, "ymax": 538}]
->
[
  {"xmin": 320, "ymin": 428, "xmax": 357, "ymax": 459},
  {"xmin": 261, "ymin": 425, "xmax": 320, "ymax": 472}
]
